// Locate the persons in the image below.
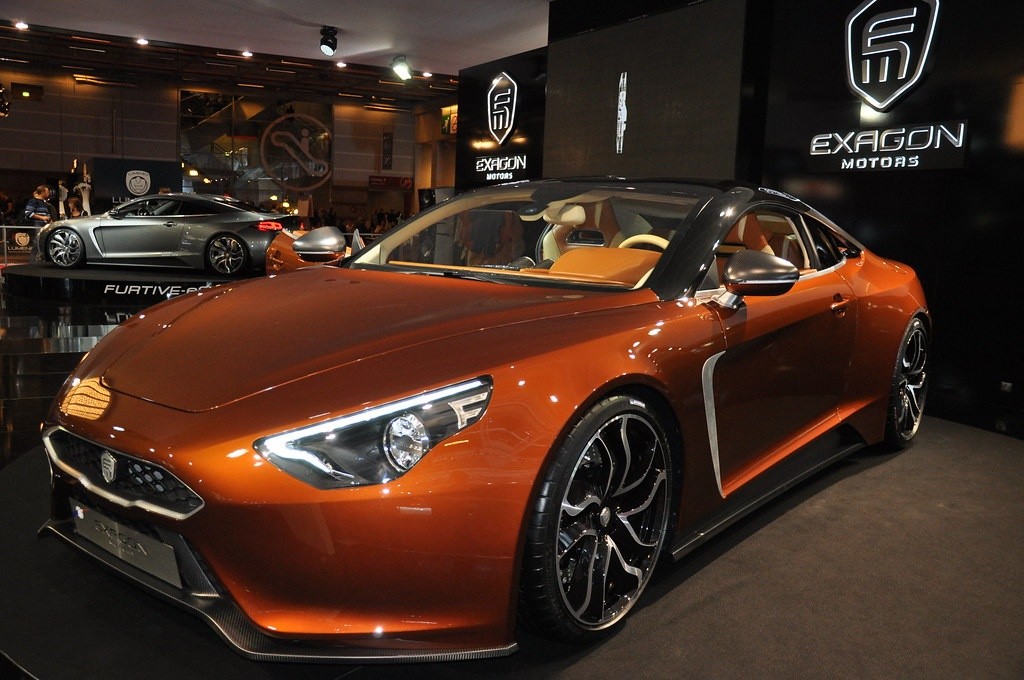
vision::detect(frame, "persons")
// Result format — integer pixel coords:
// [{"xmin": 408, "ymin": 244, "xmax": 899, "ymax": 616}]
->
[
  {"xmin": 0, "ymin": 185, "xmax": 88, "ymax": 264},
  {"xmin": 315, "ymin": 206, "xmax": 405, "ymax": 233},
  {"xmin": 259, "ymin": 202, "xmax": 291, "ymax": 214},
  {"xmin": 156, "ymin": 186, "xmax": 171, "ymax": 206}
]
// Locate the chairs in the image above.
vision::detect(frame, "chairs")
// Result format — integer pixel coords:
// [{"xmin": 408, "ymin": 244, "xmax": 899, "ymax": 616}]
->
[
  {"xmin": 541, "ymin": 200, "xmax": 625, "ymax": 269},
  {"xmin": 713, "ymin": 208, "xmax": 775, "ymax": 287}
]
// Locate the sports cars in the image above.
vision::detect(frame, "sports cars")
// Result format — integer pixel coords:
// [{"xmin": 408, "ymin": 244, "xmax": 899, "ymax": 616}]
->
[
  {"xmin": 35, "ymin": 177, "xmax": 934, "ymax": 664},
  {"xmin": 37, "ymin": 191, "xmax": 300, "ymax": 279}
]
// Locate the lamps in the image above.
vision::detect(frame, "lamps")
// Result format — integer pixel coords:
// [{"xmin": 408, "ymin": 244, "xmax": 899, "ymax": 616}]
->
[
  {"xmin": 389, "ymin": 54, "xmax": 415, "ymax": 82},
  {"xmin": 319, "ymin": 27, "xmax": 338, "ymax": 56}
]
